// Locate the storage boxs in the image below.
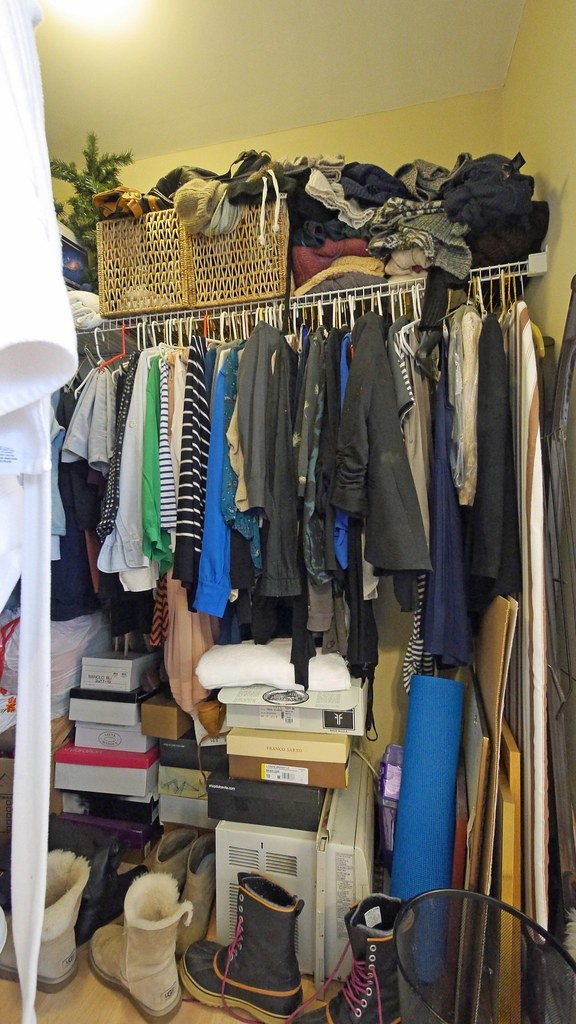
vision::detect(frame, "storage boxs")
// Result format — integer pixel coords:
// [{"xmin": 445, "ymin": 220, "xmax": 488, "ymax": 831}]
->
[{"xmin": 53, "ymin": 649, "xmax": 369, "ymax": 865}]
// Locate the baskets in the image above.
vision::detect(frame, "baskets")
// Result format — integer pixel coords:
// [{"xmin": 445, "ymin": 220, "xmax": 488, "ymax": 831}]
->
[{"xmin": 94, "ymin": 196, "xmax": 289, "ymax": 321}]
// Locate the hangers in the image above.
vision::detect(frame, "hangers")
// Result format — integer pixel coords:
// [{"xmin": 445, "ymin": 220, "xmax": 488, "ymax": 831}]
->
[{"xmin": 65, "ymin": 267, "xmax": 534, "ymax": 399}]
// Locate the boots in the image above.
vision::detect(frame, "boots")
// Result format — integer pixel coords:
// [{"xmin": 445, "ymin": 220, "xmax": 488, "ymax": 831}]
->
[
  {"xmin": 152, "ymin": 825, "xmax": 199, "ymax": 891},
  {"xmin": 65, "ymin": 823, "xmax": 150, "ymax": 947},
  {"xmin": 179, "ymin": 871, "xmax": 304, "ymax": 1024},
  {"xmin": 48, "ymin": 813, "xmax": 102, "ymax": 862},
  {"xmin": 284, "ymin": 894, "xmax": 416, "ymax": 1024},
  {"xmin": 89, "ymin": 873, "xmax": 193, "ymax": 1024},
  {"xmin": 175, "ymin": 831, "xmax": 216, "ymax": 955},
  {"xmin": 0, "ymin": 848, "xmax": 92, "ymax": 993}
]
